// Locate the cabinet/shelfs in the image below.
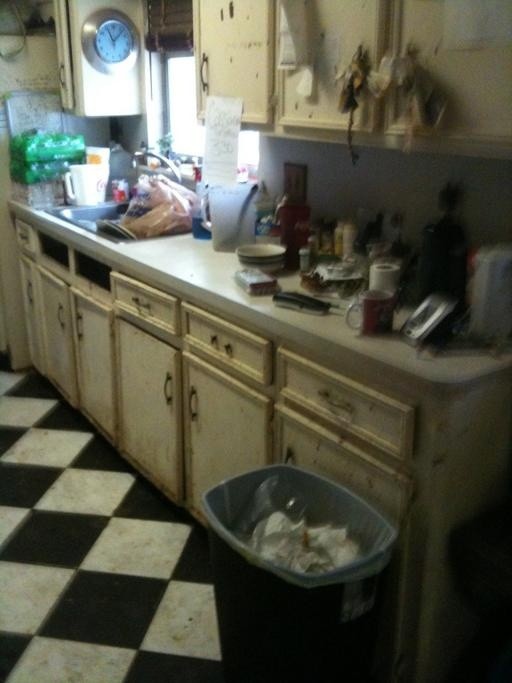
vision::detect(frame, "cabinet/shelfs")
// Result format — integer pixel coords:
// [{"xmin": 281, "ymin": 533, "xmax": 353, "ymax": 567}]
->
[
  {"xmin": 11, "ymin": 218, "xmax": 115, "ymax": 431},
  {"xmin": 275, "ymin": 302, "xmax": 510, "ymax": 679},
  {"xmin": 107, "ymin": 231, "xmax": 271, "ymax": 527},
  {"xmin": 278, "ymin": 5, "xmax": 512, "ymax": 157},
  {"xmin": 196, "ymin": 4, "xmax": 277, "ymax": 131},
  {"xmin": 51, "ymin": 0, "xmax": 148, "ymax": 118}
]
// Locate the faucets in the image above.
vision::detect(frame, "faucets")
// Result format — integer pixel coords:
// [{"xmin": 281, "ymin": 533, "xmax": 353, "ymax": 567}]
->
[{"xmin": 144, "ymin": 148, "xmax": 183, "ymax": 185}]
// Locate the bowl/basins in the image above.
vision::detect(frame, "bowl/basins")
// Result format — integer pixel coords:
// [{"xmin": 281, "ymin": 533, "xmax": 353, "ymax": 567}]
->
[{"xmin": 236, "ymin": 243, "xmax": 287, "ymax": 274}]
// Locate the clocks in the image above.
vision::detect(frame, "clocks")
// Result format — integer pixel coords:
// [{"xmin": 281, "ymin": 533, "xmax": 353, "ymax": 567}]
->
[{"xmin": 83, "ymin": 8, "xmax": 142, "ymax": 75}]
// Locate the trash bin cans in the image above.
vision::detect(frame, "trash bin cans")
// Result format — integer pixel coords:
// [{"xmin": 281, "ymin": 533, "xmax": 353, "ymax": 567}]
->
[{"xmin": 201, "ymin": 464, "xmax": 399, "ymax": 682}]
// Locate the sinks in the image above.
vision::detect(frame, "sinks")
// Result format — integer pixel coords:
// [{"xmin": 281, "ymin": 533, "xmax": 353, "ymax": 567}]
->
[{"xmin": 42, "ymin": 198, "xmax": 192, "ymax": 243}]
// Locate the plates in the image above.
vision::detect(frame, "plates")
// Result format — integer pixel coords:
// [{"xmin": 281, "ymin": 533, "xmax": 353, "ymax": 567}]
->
[{"xmin": 94, "ymin": 217, "xmax": 138, "ymax": 241}]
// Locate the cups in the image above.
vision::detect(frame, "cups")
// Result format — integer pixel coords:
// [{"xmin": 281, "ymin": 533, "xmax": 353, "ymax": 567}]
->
[
  {"xmin": 344, "ymin": 290, "xmax": 395, "ymax": 335},
  {"xmin": 278, "ymin": 205, "xmax": 311, "ymax": 271},
  {"xmin": 64, "ymin": 160, "xmax": 109, "ymax": 208}
]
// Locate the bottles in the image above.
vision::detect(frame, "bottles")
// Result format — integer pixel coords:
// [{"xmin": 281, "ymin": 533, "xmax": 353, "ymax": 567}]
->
[{"xmin": 332, "ymin": 218, "xmax": 357, "ymax": 260}]
